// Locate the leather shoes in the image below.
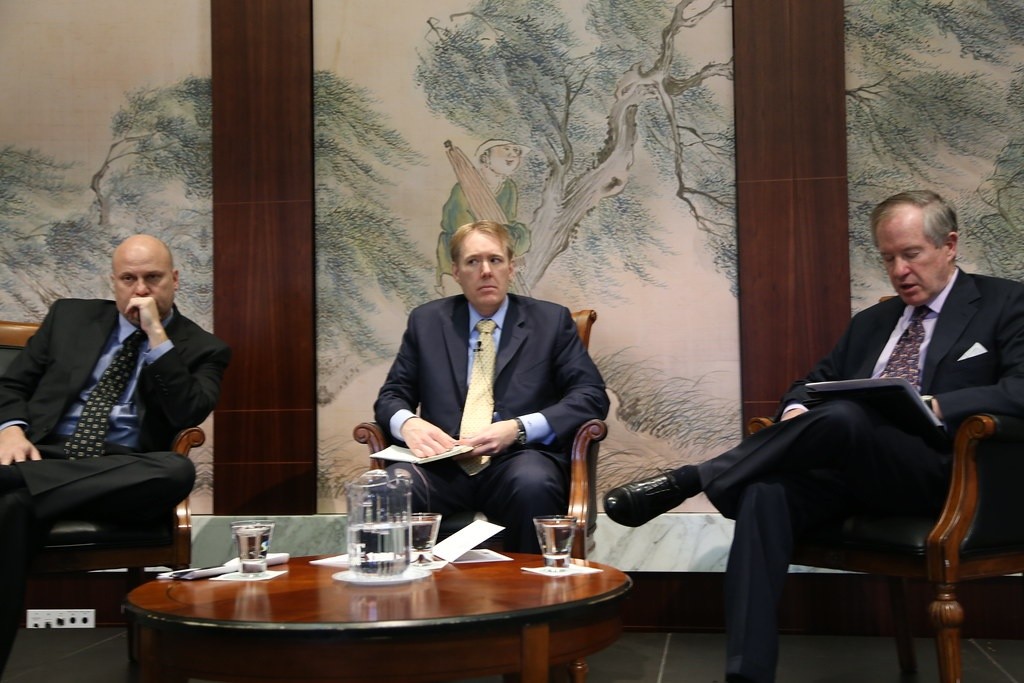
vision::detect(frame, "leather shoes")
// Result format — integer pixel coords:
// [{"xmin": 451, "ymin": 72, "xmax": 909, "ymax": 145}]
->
[{"xmin": 604, "ymin": 467, "xmax": 685, "ymax": 527}]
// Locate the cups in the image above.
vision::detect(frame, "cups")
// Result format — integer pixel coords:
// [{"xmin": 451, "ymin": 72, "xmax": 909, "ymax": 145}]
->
[
  {"xmin": 405, "ymin": 514, "xmax": 441, "ymax": 556},
  {"xmin": 533, "ymin": 515, "xmax": 577, "ymax": 571},
  {"xmin": 230, "ymin": 519, "xmax": 275, "ymax": 577}
]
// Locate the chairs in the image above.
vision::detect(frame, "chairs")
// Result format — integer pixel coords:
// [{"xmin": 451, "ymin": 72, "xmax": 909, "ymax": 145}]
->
[
  {"xmin": 750, "ymin": 294, "xmax": 1024, "ymax": 683},
  {"xmin": 0, "ymin": 319, "xmax": 206, "ymax": 572},
  {"xmin": 355, "ymin": 308, "xmax": 607, "ymax": 683}
]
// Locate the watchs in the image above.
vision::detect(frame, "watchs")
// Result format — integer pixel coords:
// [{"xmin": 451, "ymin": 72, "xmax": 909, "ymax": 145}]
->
[{"xmin": 513, "ymin": 416, "xmax": 529, "ymax": 447}]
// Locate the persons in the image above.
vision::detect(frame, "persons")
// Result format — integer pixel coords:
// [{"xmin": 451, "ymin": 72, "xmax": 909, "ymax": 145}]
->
[
  {"xmin": 364, "ymin": 221, "xmax": 610, "ymax": 553},
  {"xmin": 0, "ymin": 234, "xmax": 231, "ymax": 682},
  {"xmin": 603, "ymin": 189, "xmax": 1024, "ymax": 683}
]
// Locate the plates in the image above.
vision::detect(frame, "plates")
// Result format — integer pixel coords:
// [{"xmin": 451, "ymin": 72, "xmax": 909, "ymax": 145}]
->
[{"xmin": 332, "ymin": 568, "xmax": 432, "ymax": 585}]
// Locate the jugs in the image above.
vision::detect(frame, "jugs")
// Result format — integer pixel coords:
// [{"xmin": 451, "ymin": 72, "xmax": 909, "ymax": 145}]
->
[{"xmin": 346, "ymin": 470, "xmax": 412, "ymax": 579}]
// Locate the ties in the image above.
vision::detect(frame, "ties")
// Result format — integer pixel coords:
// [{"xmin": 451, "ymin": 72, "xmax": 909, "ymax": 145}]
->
[
  {"xmin": 879, "ymin": 305, "xmax": 932, "ymax": 393},
  {"xmin": 65, "ymin": 329, "xmax": 144, "ymax": 460},
  {"xmin": 454, "ymin": 319, "xmax": 497, "ymax": 476}
]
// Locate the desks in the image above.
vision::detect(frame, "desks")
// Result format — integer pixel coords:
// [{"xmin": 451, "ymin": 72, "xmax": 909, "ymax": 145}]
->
[{"xmin": 121, "ymin": 553, "xmax": 632, "ymax": 683}]
